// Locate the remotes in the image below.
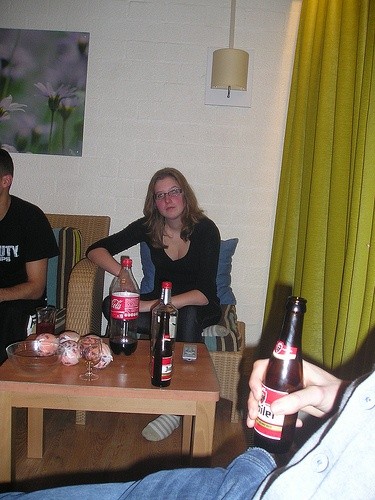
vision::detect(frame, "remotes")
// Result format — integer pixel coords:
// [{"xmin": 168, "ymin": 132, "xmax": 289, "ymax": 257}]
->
[{"xmin": 182, "ymin": 344, "xmax": 197, "ymax": 361}]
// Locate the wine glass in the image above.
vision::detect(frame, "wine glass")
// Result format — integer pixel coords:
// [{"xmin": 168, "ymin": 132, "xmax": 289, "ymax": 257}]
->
[{"xmin": 77, "ymin": 336, "xmax": 102, "ymax": 381}]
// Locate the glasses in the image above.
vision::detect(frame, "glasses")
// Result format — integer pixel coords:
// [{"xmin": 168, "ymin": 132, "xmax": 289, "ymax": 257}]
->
[{"xmin": 154, "ymin": 189, "xmax": 183, "ymax": 200}]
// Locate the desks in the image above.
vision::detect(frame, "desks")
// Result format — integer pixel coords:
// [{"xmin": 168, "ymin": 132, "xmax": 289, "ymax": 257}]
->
[{"xmin": 0, "ymin": 334, "xmax": 219, "ymax": 486}]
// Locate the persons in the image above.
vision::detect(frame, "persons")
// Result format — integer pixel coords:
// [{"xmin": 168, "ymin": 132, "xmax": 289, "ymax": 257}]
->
[
  {"xmin": 0, "ymin": 357, "xmax": 375, "ymax": 500},
  {"xmin": 86, "ymin": 168, "xmax": 222, "ymax": 441},
  {"xmin": 0, "ymin": 149, "xmax": 59, "ymax": 366}
]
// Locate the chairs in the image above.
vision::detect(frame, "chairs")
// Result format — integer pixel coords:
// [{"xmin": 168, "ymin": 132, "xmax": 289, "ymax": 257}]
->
[
  {"xmin": 44, "ymin": 215, "xmax": 110, "ymax": 426},
  {"xmin": 139, "ymin": 238, "xmax": 246, "ymax": 423}
]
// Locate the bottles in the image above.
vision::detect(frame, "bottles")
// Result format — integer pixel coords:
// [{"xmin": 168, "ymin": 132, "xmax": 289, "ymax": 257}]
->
[
  {"xmin": 251, "ymin": 296, "xmax": 307, "ymax": 454},
  {"xmin": 151, "ymin": 282, "xmax": 179, "ymax": 388},
  {"xmin": 110, "ymin": 258, "xmax": 139, "ymax": 355}
]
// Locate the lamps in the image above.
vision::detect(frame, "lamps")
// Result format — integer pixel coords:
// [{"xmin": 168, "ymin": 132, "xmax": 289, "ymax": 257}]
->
[{"xmin": 210, "ymin": 49, "xmax": 249, "ymax": 98}]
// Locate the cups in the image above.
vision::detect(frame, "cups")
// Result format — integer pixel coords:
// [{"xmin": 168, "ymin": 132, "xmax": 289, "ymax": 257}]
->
[{"xmin": 36, "ymin": 306, "xmax": 55, "ymax": 335}]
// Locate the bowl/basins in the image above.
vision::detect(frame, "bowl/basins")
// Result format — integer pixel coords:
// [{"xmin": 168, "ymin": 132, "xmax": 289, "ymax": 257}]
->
[{"xmin": 6, "ymin": 340, "xmax": 65, "ymax": 376}]
